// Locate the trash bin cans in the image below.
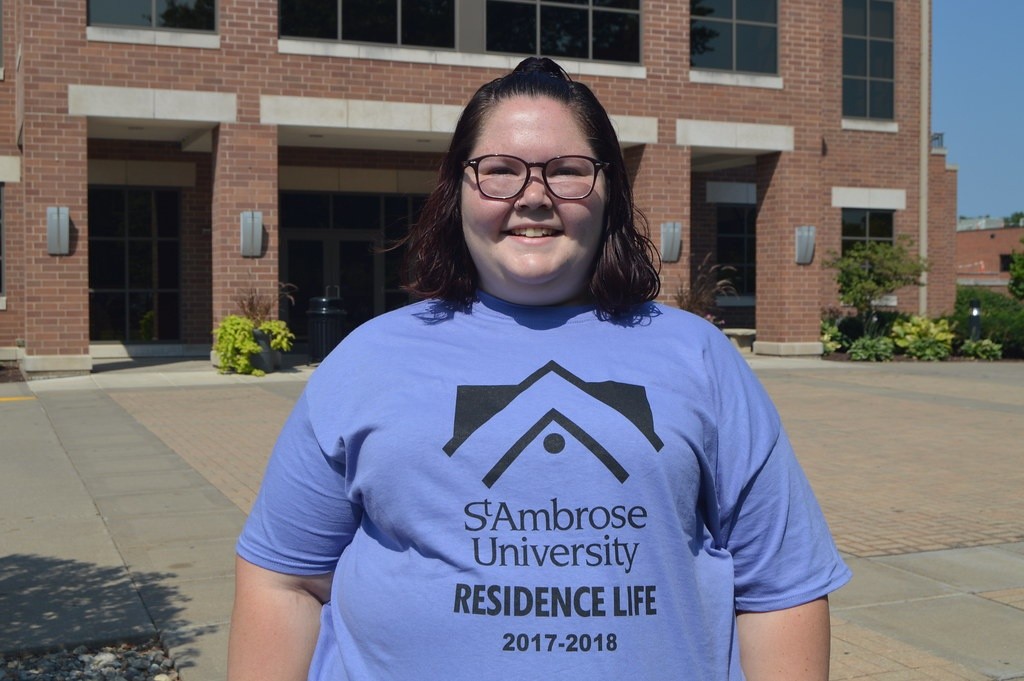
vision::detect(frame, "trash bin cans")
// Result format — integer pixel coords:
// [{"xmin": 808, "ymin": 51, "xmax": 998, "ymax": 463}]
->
[{"xmin": 305, "ymin": 296, "xmax": 342, "ymax": 366}]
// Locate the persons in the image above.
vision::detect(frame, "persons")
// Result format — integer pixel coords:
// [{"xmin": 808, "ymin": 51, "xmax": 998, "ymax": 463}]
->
[{"xmin": 227, "ymin": 56, "xmax": 853, "ymax": 681}]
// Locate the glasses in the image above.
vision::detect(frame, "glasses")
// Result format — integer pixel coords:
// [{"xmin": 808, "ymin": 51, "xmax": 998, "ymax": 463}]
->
[{"xmin": 462, "ymin": 154, "xmax": 609, "ymax": 200}]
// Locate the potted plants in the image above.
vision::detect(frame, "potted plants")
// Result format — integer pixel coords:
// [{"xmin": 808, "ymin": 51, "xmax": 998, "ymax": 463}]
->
[{"xmin": 208, "ymin": 313, "xmax": 295, "ymax": 378}]
